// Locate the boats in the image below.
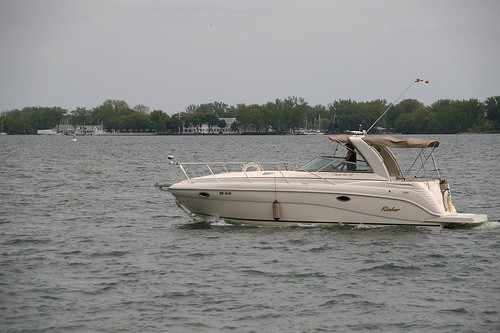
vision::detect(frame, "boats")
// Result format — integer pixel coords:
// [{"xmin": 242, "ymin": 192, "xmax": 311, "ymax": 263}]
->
[{"xmin": 154, "ymin": 128, "xmax": 489, "ymax": 227}]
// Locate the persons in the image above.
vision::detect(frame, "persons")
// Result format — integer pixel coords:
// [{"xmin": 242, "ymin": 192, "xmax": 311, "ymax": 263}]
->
[{"xmin": 346, "ymin": 142, "xmax": 356, "ymax": 171}]
